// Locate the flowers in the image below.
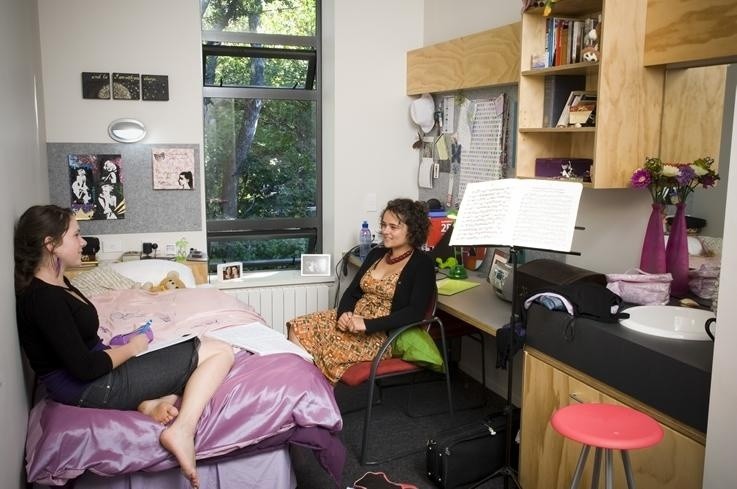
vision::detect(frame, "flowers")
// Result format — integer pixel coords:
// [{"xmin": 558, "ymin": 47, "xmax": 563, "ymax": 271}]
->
[{"xmin": 630, "ymin": 155, "xmax": 681, "ymax": 205}]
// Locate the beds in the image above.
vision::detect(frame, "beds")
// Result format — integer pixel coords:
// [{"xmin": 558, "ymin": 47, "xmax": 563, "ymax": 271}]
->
[{"xmin": 23, "ymin": 260, "xmax": 351, "ymax": 488}]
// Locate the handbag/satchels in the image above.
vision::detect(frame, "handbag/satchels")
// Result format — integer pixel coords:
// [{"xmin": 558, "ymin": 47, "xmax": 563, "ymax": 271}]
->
[
  {"xmin": 425, "ymin": 408, "xmax": 521, "ymax": 488},
  {"xmin": 605, "ymin": 268, "xmax": 674, "ymax": 305}
]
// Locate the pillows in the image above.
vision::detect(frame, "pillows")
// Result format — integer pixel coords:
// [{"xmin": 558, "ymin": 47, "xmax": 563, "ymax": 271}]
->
[{"xmin": 97, "ymin": 259, "xmax": 196, "ymax": 293}]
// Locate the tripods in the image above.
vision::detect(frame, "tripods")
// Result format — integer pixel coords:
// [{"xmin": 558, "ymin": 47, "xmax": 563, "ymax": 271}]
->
[{"xmin": 469, "ymin": 253, "xmax": 521, "ymax": 489}]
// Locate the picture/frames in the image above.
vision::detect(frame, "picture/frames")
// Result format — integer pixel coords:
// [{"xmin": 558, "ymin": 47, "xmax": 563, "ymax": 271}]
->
[
  {"xmin": 300, "ymin": 252, "xmax": 331, "ymax": 279},
  {"xmin": 216, "ymin": 262, "xmax": 244, "ymax": 284}
]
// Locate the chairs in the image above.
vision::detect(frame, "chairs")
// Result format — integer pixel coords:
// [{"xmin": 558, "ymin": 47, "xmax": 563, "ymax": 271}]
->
[{"xmin": 338, "ymin": 278, "xmax": 455, "ymax": 467}]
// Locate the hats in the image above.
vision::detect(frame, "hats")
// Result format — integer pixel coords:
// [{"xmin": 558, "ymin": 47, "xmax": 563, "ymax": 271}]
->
[{"xmin": 409, "ymin": 93, "xmax": 434, "ymax": 134}]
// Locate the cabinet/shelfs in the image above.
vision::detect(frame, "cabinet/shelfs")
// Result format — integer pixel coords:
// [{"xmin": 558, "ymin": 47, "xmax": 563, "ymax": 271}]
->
[
  {"xmin": 509, "ymin": 0, "xmax": 728, "ymax": 191},
  {"xmin": 517, "ymin": 352, "xmax": 707, "ymax": 489}
]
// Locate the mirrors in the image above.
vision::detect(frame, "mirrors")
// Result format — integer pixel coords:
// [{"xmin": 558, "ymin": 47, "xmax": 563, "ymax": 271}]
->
[{"xmin": 659, "ymin": 60, "xmax": 736, "ymax": 310}]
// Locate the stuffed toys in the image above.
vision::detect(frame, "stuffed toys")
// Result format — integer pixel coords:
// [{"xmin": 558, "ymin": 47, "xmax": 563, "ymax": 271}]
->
[{"xmin": 133, "ymin": 271, "xmax": 186, "ymax": 292}]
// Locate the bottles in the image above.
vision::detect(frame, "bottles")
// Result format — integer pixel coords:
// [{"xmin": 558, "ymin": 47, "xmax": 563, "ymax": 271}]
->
[{"xmin": 359, "ymin": 220, "xmax": 372, "ymax": 261}]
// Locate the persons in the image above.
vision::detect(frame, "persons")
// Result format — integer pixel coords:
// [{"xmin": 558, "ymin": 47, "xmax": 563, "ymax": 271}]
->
[
  {"xmin": 222, "ymin": 266, "xmax": 232, "ymax": 280},
  {"xmin": 231, "ymin": 266, "xmax": 239, "ymax": 278},
  {"xmin": 11, "ymin": 204, "xmax": 237, "ymax": 489},
  {"xmin": 284, "ymin": 198, "xmax": 438, "ymax": 395},
  {"xmin": 177, "ymin": 171, "xmax": 193, "ymax": 188},
  {"xmin": 67, "ymin": 157, "xmax": 119, "ymax": 220}
]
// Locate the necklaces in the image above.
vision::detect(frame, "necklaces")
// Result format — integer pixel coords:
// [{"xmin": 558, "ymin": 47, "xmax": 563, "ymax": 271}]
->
[{"xmin": 384, "ymin": 248, "xmax": 412, "ymax": 265}]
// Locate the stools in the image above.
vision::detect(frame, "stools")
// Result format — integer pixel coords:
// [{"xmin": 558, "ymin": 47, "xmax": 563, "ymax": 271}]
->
[
  {"xmin": 549, "ymin": 399, "xmax": 665, "ymax": 488},
  {"xmin": 672, "ymin": 156, "xmax": 722, "ymax": 205},
  {"xmin": 402, "ymin": 315, "xmax": 487, "ymax": 419}
]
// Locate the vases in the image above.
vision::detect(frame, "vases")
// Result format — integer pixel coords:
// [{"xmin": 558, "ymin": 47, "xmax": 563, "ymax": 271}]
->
[
  {"xmin": 639, "ymin": 200, "xmax": 668, "ymax": 276},
  {"xmin": 665, "ymin": 202, "xmax": 692, "ymax": 300}
]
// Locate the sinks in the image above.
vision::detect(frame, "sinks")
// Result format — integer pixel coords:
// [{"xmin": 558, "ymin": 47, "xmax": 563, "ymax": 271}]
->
[{"xmin": 617, "ymin": 305, "xmax": 716, "ymax": 342}]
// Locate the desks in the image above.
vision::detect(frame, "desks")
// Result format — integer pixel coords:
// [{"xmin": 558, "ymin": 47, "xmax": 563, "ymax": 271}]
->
[{"xmin": 338, "ymin": 243, "xmax": 528, "ymax": 413}]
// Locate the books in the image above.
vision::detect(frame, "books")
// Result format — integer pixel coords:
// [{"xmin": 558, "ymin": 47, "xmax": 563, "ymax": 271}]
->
[
  {"xmin": 447, "ymin": 177, "xmax": 584, "ymax": 253},
  {"xmin": 543, "ymin": 17, "xmax": 598, "ymax": 129}
]
